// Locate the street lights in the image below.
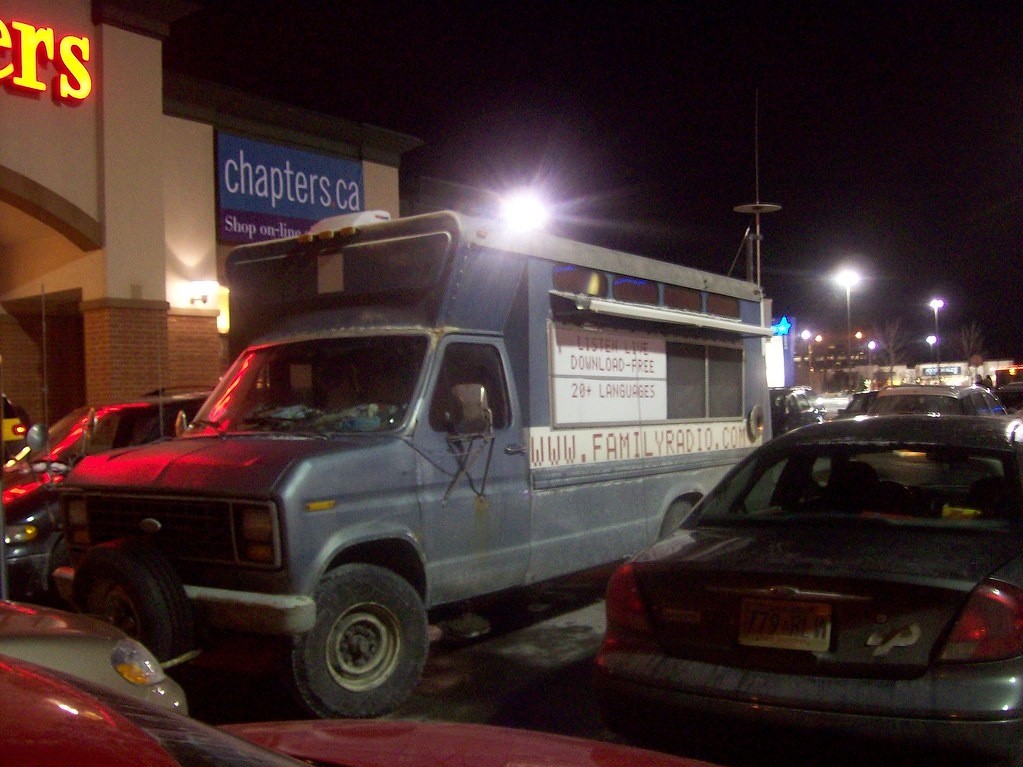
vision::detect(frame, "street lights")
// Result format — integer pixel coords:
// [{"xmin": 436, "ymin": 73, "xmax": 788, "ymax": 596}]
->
[
  {"xmin": 835, "ymin": 266, "xmax": 856, "ymax": 391},
  {"xmin": 929, "ymin": 298, "xmax": 944, "ymax": 376},
  {"xmin": 802, "ymin": 329, "xmax": 823, "ymax": 396},
  {"xmin": 866, "ymin": 340, "xmax": 878, "ymax": 389}
]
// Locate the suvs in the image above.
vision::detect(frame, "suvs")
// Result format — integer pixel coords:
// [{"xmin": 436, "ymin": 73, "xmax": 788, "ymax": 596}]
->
[{"xmin": 0, "ymin": 384, "xmax": 294, "ymax": 616}]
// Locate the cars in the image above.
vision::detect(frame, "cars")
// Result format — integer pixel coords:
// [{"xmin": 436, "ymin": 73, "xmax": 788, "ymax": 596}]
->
[
  {"xmin": 0, "ymin": 654, "xmax": 729, "ymax": 767},
  {"xmin": 769, "ymin": 386, "xmax": 828, "ymax": 434},
  {"xmin": 0, "ymin": 599, "xmax": 191, "ymax": 719},
  {"xmin": 836, "ymin": 383, "xmax": 1023, "ymax": 427},
  {"xmin": 588, "ymin": 410, "xmax": 1022, "ymax": 767}
]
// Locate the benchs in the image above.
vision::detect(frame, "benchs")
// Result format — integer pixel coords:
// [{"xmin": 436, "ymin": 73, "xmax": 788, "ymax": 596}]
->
[{"xmin": 781, "ymin": 462, "xmax": 1009, "ymax": 518}]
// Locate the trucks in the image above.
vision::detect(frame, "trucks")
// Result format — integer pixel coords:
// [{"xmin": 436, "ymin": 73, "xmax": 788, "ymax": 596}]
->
[{"xmin": 26, "ymin": 208, "xmax": 775, "ymax": 724}]
